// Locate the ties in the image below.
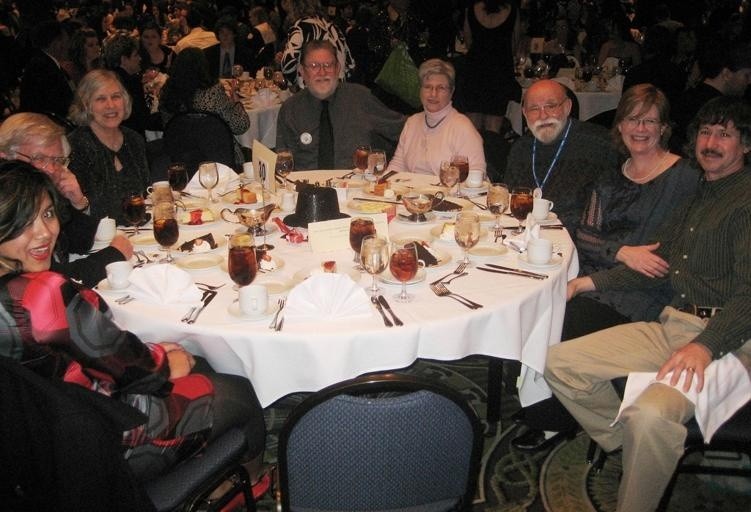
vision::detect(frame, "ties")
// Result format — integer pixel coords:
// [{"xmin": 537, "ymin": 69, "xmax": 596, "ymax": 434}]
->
[
  {"xmin": 222, "ymin": 52, "xmax": 231, "ymax": 77},
  {"xmin": 318, "ymin": 99, "xmax": 334, "ymax": 169}
]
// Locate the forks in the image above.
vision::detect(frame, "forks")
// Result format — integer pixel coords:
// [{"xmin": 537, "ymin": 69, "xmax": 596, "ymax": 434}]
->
[
  {"xmin": 427, "ymin": 259, "xmax": 484, "ymax": 312},
  {"xmin": 270, "ymin": 297, "xmax": 284, "ymax": 329}
]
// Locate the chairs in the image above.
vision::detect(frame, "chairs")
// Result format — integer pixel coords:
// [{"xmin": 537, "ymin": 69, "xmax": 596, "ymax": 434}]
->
[
  {"xmin": 584, "ymin": 375, "xmax": 751, "ymax": 512},
  {"xmin": 477, "ymin": 129, "xmax": 510, "ymax": 187},
  {"xmin": 163, "ymin": 107, "xmax": 236, "ymax": 178},
  {"xmin": 0, "ymin": 354, "xmax": 261, "ymax": 512},
  {"xmin": 269, "ymin": 370, "xmax": 485, "ymax": 511}
]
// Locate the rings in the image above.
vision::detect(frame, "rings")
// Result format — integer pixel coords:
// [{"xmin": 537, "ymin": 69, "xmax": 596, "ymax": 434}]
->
[{"xmin": 688, "ymin": 367, "xmax": 695, "ymax": 372}]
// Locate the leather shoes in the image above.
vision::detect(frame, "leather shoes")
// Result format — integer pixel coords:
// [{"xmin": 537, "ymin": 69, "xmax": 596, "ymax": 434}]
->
[
  {"xmin": 219, "ymin": 474, "xmax": 271, "ymax": 512},
  {"xmin": 512, "ymin": 414, "xmax": 565, "ymax": 452}
]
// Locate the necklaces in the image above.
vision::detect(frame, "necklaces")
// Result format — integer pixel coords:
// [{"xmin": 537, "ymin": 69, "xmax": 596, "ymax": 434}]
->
[{"xmin": 1, "ymin": 261, "xmax": 15, "ymax": 270}]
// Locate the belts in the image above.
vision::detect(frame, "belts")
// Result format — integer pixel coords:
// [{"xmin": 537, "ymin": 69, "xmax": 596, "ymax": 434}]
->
[{"xmin": 675, "ymin": 303, "xmax": 721, "ymax": 317}]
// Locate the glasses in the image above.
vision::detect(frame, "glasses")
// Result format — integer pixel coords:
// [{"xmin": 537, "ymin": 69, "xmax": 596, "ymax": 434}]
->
[
  {"xmin": 15, "ymin": 151, "xmax": 70, "ymax": 168},
  {"xmin": 304, "ymin": 63, "xmax": 336, "ymax": 70}
]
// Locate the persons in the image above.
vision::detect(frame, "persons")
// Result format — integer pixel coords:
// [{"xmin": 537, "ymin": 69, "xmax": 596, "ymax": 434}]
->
[
  {"xmin": 512, "ymin": 86, "xmax": 699, "ymax": 452},
  {"xmin": 1, "ymin": 113, "xmax": 134, "ymax": 289},
  {"xmin": 0, "ymin": 158, "xmax": 274, "ymax": 512},
  {"xmin": 544, "ymin": 96, "xmax": 749, "ymax": 510},
  {"xmin": 1, "ymin": 1, "xmax": 750, "ymax": 239}
]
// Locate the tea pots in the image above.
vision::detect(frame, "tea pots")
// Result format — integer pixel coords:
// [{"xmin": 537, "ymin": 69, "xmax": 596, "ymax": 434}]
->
[{"xmin": 220, "ymin": 204, "xmax": 281, "ymax": 235}]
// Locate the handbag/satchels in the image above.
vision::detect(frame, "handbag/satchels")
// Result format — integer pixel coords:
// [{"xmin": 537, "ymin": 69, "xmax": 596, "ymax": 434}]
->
[{"xmin": 375, "ymin": 43, "xmax": 423, "ymax": 107}]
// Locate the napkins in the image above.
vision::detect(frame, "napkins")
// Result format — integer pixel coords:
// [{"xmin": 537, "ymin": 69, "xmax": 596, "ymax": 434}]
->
[
  {"xmin": 126, "ymin": 263, "xmax": 205, "ymax": 314},
  {"xmin": 504, "ymin": 212, "xmax": 540, "ymax": 253},
  {"xmin": 182, "ymin": 162, "xmax": 244, "ymax": 200},
  {"xmin": 284, "ymin": 272, "xmax": 371, "ymax": 323}
]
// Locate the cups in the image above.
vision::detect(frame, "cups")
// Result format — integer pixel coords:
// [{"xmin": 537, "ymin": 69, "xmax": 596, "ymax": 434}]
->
[
  {"xmin": 104, "ymin": 261, "xmax": 132, "ymax": 288},
  {"xmin": 243, "ymin": 162, "xmax": 257, "ymax": 178},
  {"xmin": 464, "ymin": 169, "xmax": 484, "ymax": 187},
  {"xmin": 526, "ymin": 240, "xmax": 557, "ymax": 264},
  {"xmin": 227, "ymin": 232, "xmax": 268, "ymax": 315},
  {"xmin": 530, "ymin": 199, "xmax": 554, "ymax": 220}
]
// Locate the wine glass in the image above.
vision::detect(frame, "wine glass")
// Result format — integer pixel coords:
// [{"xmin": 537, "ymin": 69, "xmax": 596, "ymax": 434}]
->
[
  {"xmin": 124, "ymin": 161, "xmax": 219, "ymax": 259},
  {"xmin": 227, "ymin": 63, "xmax": 289, "ymax": 107},
  {"xmin": 454, "ymin": 180, "xmax": 532, "ymax": 263},
  {"xmin": 269, "ymin": 146, "xmax": 468, "ymax": 194},
  {"xmin": 346, "ymin": 215, "xmax": 418, "ymax": 306}
]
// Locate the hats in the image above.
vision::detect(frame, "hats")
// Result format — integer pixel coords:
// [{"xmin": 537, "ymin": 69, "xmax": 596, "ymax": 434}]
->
[{"xmin": 283, "ymin": 187, "xmax": 350, "ymax": 228}]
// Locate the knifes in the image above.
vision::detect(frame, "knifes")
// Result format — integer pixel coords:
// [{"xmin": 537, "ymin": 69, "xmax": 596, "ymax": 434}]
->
[
  {"xmin": 372, "ymin": 295, "xmax": 402, "ymax": 328},
  {"xmin": 476, "ymin": 260, "xmax": 549, "ymax": 284},
  {"xmin": 181, "ymin": 288, "xmax": 215, "ymax": 324}
]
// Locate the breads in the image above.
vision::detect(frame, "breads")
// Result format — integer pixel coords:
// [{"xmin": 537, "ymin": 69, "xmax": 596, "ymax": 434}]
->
[
  {"xmin": 182, "ymin": 207, "xmax": 214, "ymax": 224},
  {"xmin": 374, "ymin": 181, "xmax": 390, "ymax": 197},
  {"xmin": 235, "ymin": 188, "xmax": 257, "ymax": 204}
]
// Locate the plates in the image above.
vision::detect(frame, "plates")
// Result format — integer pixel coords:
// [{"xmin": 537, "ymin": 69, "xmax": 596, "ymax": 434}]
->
[
  {"xmin": 168, "ymin": 254, "xmax": 223, "ymax": 271},
  {"xmin": 521, "ymin": 256, "xmax": 561, "ymax": 272},
  {"xmin": 97, "ymin": 279, "xmax": 132, "ymax": 293}
]
